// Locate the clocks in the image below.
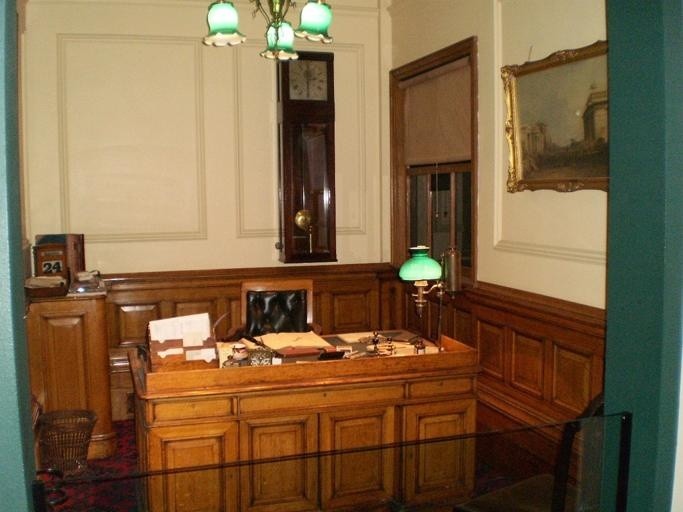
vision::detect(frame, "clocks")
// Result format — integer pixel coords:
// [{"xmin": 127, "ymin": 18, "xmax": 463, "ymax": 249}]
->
[{"xmin": 275, "ymin": 51, "xmax": 337, "ymax": 263}]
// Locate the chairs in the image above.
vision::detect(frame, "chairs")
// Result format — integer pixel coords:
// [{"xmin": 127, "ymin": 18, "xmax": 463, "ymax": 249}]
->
[
  {"xmin": 453, "ymin": 391, "xmax": 604, "ymax": 512},
  {"xmin": 212, "ymin": 278, "xmax": 322, "ymax": 341}
]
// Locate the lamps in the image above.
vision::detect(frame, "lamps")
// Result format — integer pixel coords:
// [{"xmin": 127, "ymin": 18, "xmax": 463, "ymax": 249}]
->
[
  {"xmin": 203, "ymin": 0, "xmax": 334, "ymax": 61},
  {"xmin": 399, "ymin": 246, "xmax": 462, "ymax": 352}
]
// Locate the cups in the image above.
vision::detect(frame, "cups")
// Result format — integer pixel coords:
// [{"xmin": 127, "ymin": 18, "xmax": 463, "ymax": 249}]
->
[{"xmin": 231, "ymin": 344, "xmax": 248, "ymax": 362}]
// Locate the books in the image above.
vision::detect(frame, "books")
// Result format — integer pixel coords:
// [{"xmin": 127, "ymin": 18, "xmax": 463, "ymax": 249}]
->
[
  {"xmin": 32, "ymin": 242, "xmax": 69, "ymax": 277},
  {"xmin": 35, "ymin": 233, "xmax": 86, "ymax": 284}
]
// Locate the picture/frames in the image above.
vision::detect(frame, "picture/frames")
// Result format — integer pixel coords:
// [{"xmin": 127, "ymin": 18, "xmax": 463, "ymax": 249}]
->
[{"xmin": 501, "ymin": 39, "xmax": 609, "ymax": 194}]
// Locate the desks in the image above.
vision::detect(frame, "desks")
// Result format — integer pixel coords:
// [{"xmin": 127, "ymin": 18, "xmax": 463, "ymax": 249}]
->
[{"xmin": 127, "ymin": 330, "xmax": 484, "ymax": 512}]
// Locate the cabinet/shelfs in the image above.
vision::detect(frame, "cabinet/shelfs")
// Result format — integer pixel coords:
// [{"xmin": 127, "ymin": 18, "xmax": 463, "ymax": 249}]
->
[{"xmin": 29, "ymin": 294, "xmax": 116, "ymax": 464}]
